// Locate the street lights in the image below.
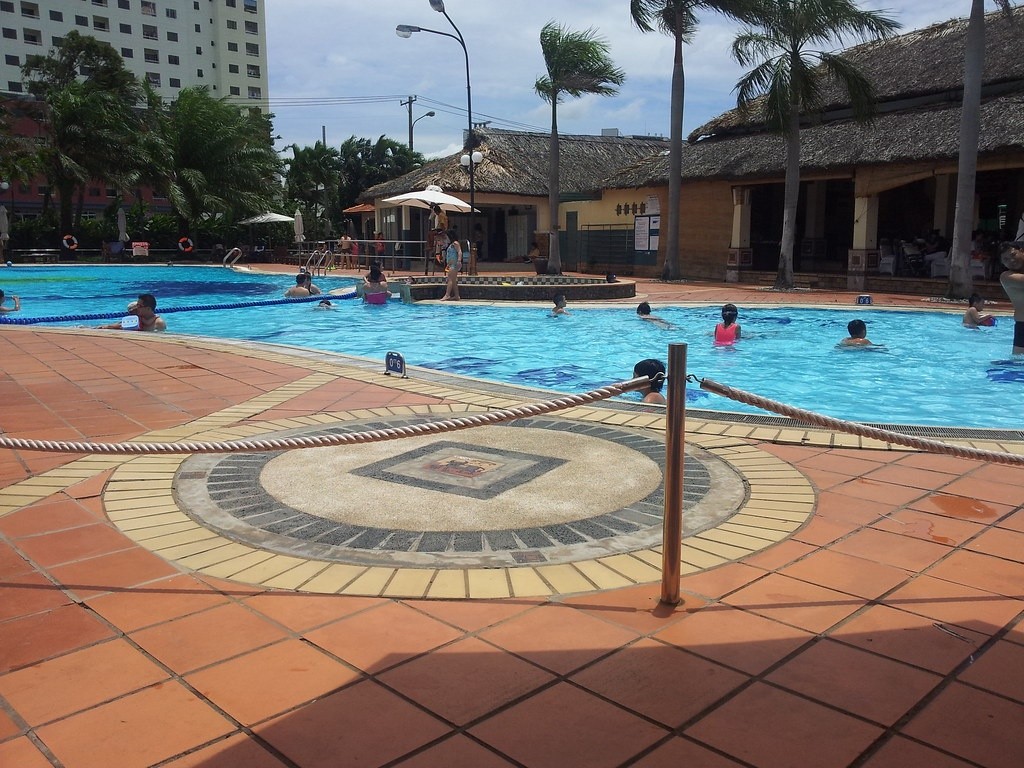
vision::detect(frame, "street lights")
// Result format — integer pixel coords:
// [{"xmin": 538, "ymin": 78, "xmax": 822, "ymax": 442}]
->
[{"xmin": 395, "ymin": 0, "xmax": 484, "ymax": 276}]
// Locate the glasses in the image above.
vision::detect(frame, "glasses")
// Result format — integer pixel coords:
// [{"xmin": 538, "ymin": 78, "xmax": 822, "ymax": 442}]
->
[{"xmin": 137, "ymin": 304, "xmax": 147, "ymax": 308}]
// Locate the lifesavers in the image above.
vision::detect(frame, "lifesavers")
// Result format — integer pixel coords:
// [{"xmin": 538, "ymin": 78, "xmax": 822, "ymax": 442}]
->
[
  {"xmin": 178, "ymin": 238, "xmax": 193, "ymax": 252},
  {"xmin": 63, "ymin": 235, "xmax": 78, "ymax": 250}
]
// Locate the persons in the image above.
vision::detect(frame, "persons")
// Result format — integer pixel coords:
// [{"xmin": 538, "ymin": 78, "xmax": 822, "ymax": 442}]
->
[
  {"xmin": 0, "ymin": 289, "xmax": 20, "ymax": 311},
  {"xmin": 963, "ymin": 293, "xmax": 994, "ymax": 325},
  {"xmin": 128, "ymin": 302, "xmax": 139, "ymax": 315},
  {"xmin": 551, "ymin": 294, "xmax": 570, "ymax": 314},
  {"xmin": 637, "ymin": 302, "xmax": 652, "ymax": 315},
  {"xmin": 714, "ymin": 304, "xmax": 741, "ymax": 340},
  {"xmin": 276, "ymin": 206, "xmax": 541, "ymax": 310},
  {"xmin": 844, "ymin": 320, "xmax": 872, "ymax": 345},
  {"xmin": 923, "ymin": 229, "xmax": 992, "ymax": 270},
  {"xmin": 95, "ymin": 293, "xmax": 166, "ymax": 332},
  {"xmin": 632, "ymin": 358, "xmax": 666, "ymax": 405},
  {"xmin": 999, "ymin": 240, "xmax": 1024, "ymax": 355}
]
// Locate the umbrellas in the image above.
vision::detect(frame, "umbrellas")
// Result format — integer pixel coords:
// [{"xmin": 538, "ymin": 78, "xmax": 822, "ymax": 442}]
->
[
  {"xmin": 294, "ymin": 209, "xmax": 306, "ymax": 269},
  {"xmin": 118, "ymin": 208, "xmax": 130, "ymax": 243},
  {"xmin": 343, "ymin": 203, "xmax": 376, "ymax": 213},
  {"xmin": 0, "ymin": 205, "xmax": 9, "ymax": 245},
  {"xmin": 237, "ymin": 211, "xmax": 295, "ymax": 263},
  {"xmin": 382, "ymin": 185, "xmax": 481, "ymax": 276}
]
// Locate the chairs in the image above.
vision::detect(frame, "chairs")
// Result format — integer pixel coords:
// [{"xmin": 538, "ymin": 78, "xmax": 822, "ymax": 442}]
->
[
  {"xmin": 880, "ymin": 240, "xmax": 992, "ymax": 279},
  {"xmin": 276, "ymin": 246, "xmax": 298, "ymax": 265},
  {"xmin": 246, "ymin": 246, "xmax": 265, "ymax": 263}
]
